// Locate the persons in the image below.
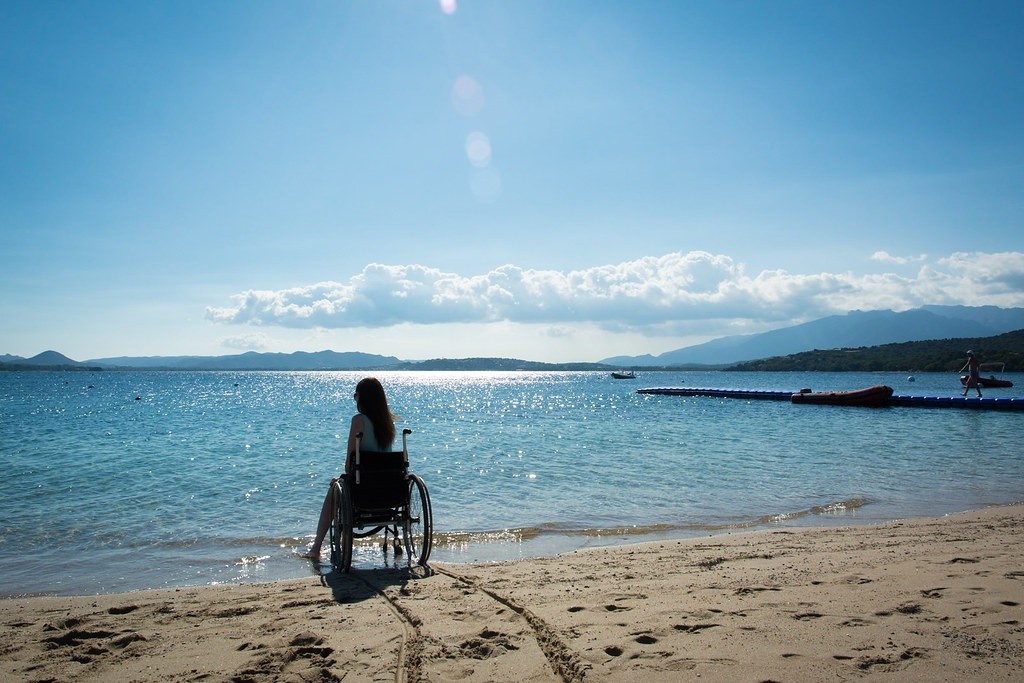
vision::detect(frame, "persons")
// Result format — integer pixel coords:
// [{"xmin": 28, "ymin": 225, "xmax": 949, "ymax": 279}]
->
[
  {"xmin": 303, "ymin": 378, "xmax": 396, "ymax": 562},
  {"xmin": 959, "ymin": 350, "xmax": 982, "ymax": 397}
]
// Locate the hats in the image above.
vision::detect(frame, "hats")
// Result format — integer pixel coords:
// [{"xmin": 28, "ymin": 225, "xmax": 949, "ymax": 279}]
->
[{"xmin": 966, "ymin": 349, "xmax": 974, "ymax": 355}]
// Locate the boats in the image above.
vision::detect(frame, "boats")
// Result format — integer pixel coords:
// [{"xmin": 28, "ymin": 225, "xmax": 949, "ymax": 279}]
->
[
  {"xmin": 791, "ymin": 386, "xmax": 893, "ymax": 406},
  {"xmin": 961, "ymin": 375, "xmax": 1014, "ymax": 387},
  {"xmin": 612, "ymin": 373, "xmax": 638, "ymax": 379}
]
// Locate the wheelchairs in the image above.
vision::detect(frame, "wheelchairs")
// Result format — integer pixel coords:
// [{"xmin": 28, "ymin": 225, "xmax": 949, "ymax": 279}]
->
[{"xmin": 329, "ymin": 428, "xmax": 434, "ymax": 574}]
